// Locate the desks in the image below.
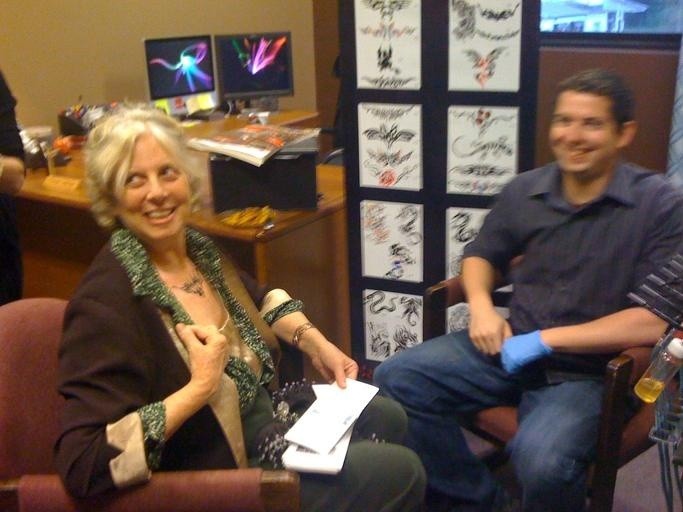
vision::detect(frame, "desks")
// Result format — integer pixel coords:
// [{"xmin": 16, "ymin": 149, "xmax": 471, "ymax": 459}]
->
[{"xmin": 20, "ymin": 109, "xmax": 352, "ymax": 377}]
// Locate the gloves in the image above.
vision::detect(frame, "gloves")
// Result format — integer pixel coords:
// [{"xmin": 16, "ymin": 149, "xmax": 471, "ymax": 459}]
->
[{"xmin": 500, "ymin": 330, "xmax": 553, "ymax": 375}]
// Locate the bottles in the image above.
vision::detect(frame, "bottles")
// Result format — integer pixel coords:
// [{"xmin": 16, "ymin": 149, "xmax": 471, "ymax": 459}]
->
[{"xmin": 633, "ymin": 338, "xmax": 683, "ymax": 405}]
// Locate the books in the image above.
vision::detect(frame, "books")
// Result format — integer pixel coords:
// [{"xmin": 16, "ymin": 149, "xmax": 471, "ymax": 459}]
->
[{"xmin": 187, "ymin": 123, "xmax": 322, "ymax": 167}]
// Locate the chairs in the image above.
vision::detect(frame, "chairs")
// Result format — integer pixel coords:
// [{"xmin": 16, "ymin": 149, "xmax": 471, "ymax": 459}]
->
[
  {"xmin": 318, "ymin": 55, "xmax": 345, "ymax": 164},
  {"xmin": 0, "ymin": 294, "xmax": 303, "ymax": 511},
  {"xmin": 424, "ymin": 255, "xmax": 681, "ymax": 511}
]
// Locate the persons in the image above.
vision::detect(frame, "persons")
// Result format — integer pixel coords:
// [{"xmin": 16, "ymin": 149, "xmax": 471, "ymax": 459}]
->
[
  {"xmin": 0, "ymin": 69, "xmax": 25, "ymax": 307},
  {"xmin": 50, "ymin": 106, "xmax": 428, "ymax": 512},
  {"xmin": 371, "ymin": 68, "xmax": 683, "ymax": 512}
]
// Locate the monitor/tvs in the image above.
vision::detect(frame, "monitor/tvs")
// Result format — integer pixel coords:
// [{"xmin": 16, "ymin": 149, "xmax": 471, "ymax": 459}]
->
[
  {"xmin": 214, "ymin": 31, "xmax": 294, "ymax": 112},
  {"xmin": 144, "ymin": 35, "xmax": 216, "ymax": 120}
]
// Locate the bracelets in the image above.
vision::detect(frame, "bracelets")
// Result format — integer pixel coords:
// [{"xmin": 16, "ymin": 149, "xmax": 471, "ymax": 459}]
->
[{"xmin": 291, "ymin": 322, "xmax": 316, "ymax": 349}]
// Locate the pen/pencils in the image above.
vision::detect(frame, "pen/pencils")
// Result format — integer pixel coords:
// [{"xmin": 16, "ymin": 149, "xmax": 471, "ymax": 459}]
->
[{"xmin": 64, "ymin": 101, "xmax": 87, "ymax": 120}]
